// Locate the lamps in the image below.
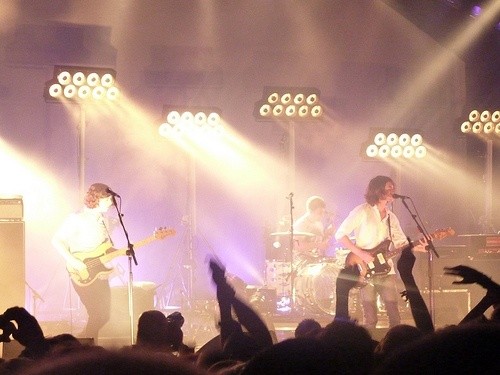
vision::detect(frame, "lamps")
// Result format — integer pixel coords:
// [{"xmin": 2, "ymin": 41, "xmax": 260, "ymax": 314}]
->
[
  {"xmin": 161, "ymin": 105, "xmax": 224, "ymax": 139},
  {"xmin": 464, "ymin": 0, "xmax": 489, "ymax": 22},
  {"xmin": 254, "ymin": 87, "xmax": 326, "ymax": 122},
  {"xmin": 456, "ymin": 102, "xmax": 500, "ymax": 141},
  {"xmin": 43, "ymin": 65, "xmax": 116, "ymax": 105},
  {"xmin": 359, "ymin": 127, "xmax": 427, "ymax": 163}
]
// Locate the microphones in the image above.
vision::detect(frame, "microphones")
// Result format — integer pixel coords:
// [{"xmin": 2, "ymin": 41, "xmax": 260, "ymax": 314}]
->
[
  {"xmin": 392, "ymin": 194, "xmax": 410, "ymax": 199},
  {"xmin": 104, "ymin": 188, "xmax": 121, "ymax": 198}
]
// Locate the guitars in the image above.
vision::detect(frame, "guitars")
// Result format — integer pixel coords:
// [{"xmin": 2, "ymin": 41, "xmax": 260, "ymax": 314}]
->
[
  {"xmin": 68, "ymin": 227, "xmax": 176, "ymax": 287},
  {"xmin": 344, "ymin": 226, "xmax": 455, "ymax": 289}
]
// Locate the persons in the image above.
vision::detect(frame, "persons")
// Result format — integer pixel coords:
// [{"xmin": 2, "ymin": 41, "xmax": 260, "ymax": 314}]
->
[
  {"xmin": 293, "ymin": 197, "xmax": 333, "ymax": 261},
  {"xmin": 335, "ymin": 175, "xmax": 433, "ymax": 332},
  {"xmin": 0, "ymin": 247, "xmax": 500, "ymax": 375},
  {"xmin": 53, "ymin": 183, "xmax": 118, "ymax": 346}
]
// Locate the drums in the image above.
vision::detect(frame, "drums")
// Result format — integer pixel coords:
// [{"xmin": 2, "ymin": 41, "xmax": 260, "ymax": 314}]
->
[
  {"xmin": 337, "ymin": 249, "xmax": 350, "ymax": 263},
  {"xmin": 293, "ymin": 264, "xmax": 357, "ymax": 317},
  {"xmin": 263, "ymin": 259, "xmax": 293, "ymax": 297}
]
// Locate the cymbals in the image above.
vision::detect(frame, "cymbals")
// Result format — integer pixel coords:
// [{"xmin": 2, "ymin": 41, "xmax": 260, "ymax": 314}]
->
[{"xmin": 271, "ymin": 230, "xmax": 315, "ymax": 238}]
